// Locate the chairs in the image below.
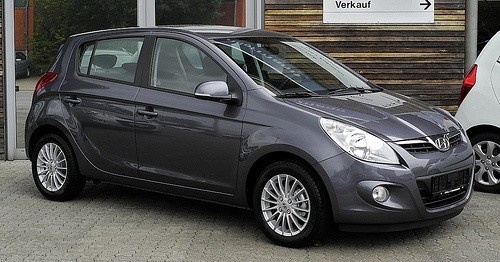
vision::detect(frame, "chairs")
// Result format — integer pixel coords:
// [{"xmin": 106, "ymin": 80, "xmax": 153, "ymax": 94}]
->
[{"xmin": 88, "ymin": 54, "xmax": 245, "ymax": 102}]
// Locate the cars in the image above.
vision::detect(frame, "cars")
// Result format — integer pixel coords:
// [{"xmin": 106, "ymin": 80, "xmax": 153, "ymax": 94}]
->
[
  {"xmin": 16, "ymin": 51, "xmax": 30, "ymax": 78},
  {"xmin": 455, "ymin": 31, "xmax": 500, "ymax": 194},
  {"xmin": 23, "ymin": 25, "xmax": 475, "ymax": 248}
]
